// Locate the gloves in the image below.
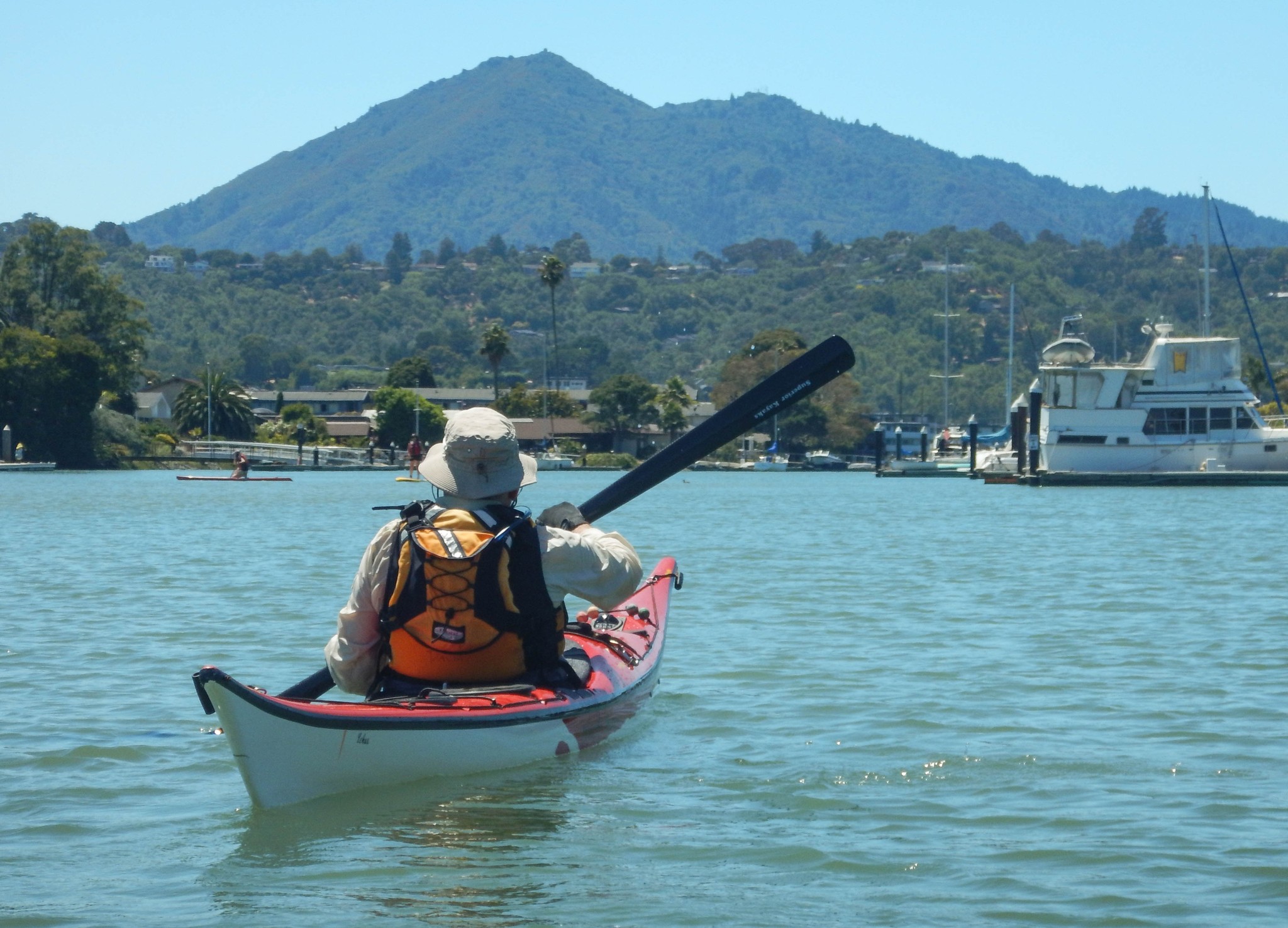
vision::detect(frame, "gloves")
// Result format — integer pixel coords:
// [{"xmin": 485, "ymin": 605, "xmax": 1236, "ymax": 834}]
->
[{"xmin": 537, "ymin": 502, "xmax": 588, "ymax": 530}]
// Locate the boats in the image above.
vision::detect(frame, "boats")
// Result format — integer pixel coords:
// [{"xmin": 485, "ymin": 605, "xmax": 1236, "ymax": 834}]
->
[
  {"xmin": 190, "ymin": 556, "xmax": 680, "ymax": 815},
  {"xmin": 806, "ymin": 452, "xmax": 851, "ymax": 472},
  {"xmin": 527, "ymin": 452, "xmax": 575, "ymax": 472},
  {"xmin": 751, "ymin": 461, "xmax": 790, "ymax": 473},
  {"xmin": 967, "ymin": 175, "xmax": 1287, "ymax": 489}
]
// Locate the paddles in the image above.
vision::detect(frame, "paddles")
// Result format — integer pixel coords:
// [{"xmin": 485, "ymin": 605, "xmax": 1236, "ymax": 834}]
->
[{"xmin": 270, "ymin": 334, "xmax": 859, "ymax": 704}]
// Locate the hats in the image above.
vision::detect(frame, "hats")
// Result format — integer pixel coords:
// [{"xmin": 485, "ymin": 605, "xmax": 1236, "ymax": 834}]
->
[
  {"xmin": 411, "ymin": 434, "xmax": 417, "ymax": 438},
  {"xmin": 418, "ymin": 408, "xmax": 537, "ymax": 498}
]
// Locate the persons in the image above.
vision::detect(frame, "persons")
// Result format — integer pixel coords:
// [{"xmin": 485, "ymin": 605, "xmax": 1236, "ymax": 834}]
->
[
  {"xmin": 407, "ymin": 434, "xmax": 425, "ymax": 479},
  {"xmin": 322, "ymin": 406, "xmax": 643, "ymax": 699},
  {"xmin": 231, "ymin": 450, "xmax": 249, "ymax": 478}
]
